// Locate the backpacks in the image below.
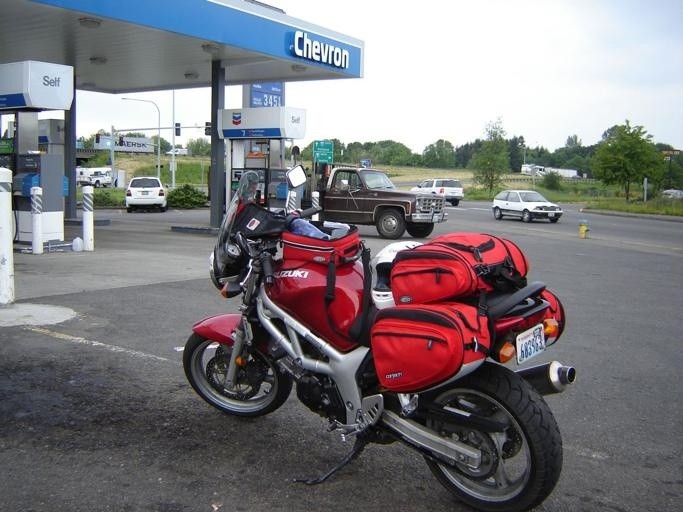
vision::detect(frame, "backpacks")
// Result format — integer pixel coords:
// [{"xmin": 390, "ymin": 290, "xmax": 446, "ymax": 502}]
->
[{"xmin": 389, "ymin": 231, "xmax": 529, "ymax": 306}]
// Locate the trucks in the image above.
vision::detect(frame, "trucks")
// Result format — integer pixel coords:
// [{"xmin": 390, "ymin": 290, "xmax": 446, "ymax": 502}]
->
[
  {"xmin": 519, "ymin": 164, "xmax": 578, "ymax": 181},
  {"xmin": 76, "ymin": 167, "xmax": 117, "ymax": 188},
  {"xmin": 165, "ymin": 148, "xmax": 188, "ymax": 156}
]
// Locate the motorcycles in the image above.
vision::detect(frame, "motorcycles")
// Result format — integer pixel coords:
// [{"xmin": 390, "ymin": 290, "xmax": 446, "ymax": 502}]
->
[{"xmin": 181, "ymin": 162, "xmax": 575, "ymax": 512}]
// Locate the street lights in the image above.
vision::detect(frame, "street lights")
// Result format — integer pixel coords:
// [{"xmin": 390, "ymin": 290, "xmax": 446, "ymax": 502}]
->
[{"xmin": 121, "ymin": 97, "xmax": 160, "ymax": 179}]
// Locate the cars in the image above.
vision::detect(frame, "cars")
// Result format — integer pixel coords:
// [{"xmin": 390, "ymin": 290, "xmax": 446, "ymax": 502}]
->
[
  {"xmin": 491, "ymin": 189, "xmax": 563, "ymax": 224},
  {"xmin": 659, "ymin": 190, "xmax": 683, "ymax": 199}
]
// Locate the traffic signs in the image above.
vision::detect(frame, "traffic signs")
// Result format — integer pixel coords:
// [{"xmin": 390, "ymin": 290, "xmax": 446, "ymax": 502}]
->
[
  {"xmin": 313, "ymin": 152, "xmax": 333, "ymax": 164},
  {"xmin": 313, "ymin": 141, "xmax": 332, "ymax": 152}
]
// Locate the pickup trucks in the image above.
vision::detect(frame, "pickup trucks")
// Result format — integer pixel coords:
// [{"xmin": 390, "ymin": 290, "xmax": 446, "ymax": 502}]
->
[{"xmin": 267, "ymin": 165, "xmax": 449, "ymax": 239}]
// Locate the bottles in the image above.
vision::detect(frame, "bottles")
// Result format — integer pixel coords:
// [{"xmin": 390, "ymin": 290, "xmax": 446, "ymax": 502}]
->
[{"xmin": 285, "ymin": 214, "xmax": 329, "ymax": 245}]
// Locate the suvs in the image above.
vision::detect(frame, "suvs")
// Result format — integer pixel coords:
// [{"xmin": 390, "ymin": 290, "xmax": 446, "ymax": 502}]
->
[
  {"xmin": 124, "ymin": 177, "xmax": 168, "ymax": 213},
  {"xmin": 409, "ymin": 178, "xmax": 465, "ymax": 207}
]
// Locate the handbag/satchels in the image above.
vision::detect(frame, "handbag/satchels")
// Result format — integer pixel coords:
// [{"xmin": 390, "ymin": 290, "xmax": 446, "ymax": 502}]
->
[
  {"xmin": 229, "ymin": 202, "xmax": 363, "ymax": 267},
  {"xmin": 369, "ymin": 300, "xmax": 492, "ymax": 394},
  {"xmin": 538, "ymin": 288, "xmax": 566, "ymax": 346}
]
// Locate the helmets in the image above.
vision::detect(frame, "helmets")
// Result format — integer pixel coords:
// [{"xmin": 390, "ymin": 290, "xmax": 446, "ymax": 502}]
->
[{"xmin": 368, "ymin": 241, "xmax": 424, "ymax": 311}]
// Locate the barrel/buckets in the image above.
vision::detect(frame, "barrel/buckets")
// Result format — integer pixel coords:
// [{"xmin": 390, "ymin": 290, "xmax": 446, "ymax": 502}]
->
[{"xmin": 72, "ymin": 237, "xmax": 83, "ymax": 252}]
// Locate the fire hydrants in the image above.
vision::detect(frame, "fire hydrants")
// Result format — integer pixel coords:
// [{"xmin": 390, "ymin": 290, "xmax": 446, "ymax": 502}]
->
[{"xmin": 578, "ymin": 219, "xmax": 589, "ymax": 240}]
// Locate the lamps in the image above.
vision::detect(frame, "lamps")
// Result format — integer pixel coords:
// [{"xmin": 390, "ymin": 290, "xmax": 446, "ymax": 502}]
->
[
  {"xmin": 77, "ymin": 17, "xmax": 103, "ymax": 30},
  {"xmin": 183, "ymin": 71, "xmax": 198, "ymax": 80},
  {"xmin": 201, "ymin": 43, "xmax": 220, "ymax": 54},
  {"xmin": 290, "ymin": 64, "xmax": 307, "ymax": 71},
  {"xmin": 87, "ymin": 57, "xmax": 107, "ymax": 65}
]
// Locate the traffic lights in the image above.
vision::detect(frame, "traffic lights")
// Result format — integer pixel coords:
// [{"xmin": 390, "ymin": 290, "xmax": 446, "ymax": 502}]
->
[
  {"xmin": 174, "ymin": 123, "xmax": 181, "ymax": 136},
  {"xmin": 95, "ymin": 134, "xmax": 100, "ymax": 143},
  {"xmin": 118, "ymin": 137, "xmax": 123, "ymax": 146}
]
